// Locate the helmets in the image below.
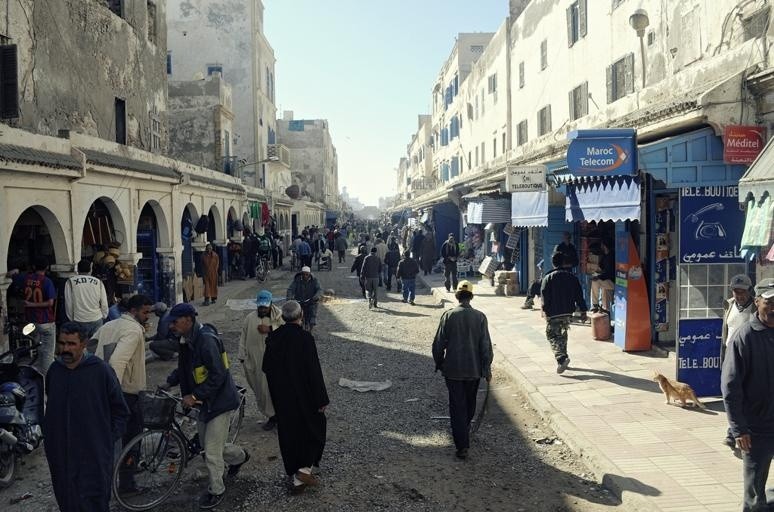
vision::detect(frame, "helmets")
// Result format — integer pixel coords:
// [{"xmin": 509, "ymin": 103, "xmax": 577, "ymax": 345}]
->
[{"xmin": 0, "ymin": 382, "xmax": 27, "ymax": 400}]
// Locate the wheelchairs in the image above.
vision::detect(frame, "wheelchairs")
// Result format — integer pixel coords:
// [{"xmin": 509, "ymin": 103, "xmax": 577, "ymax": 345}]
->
[{"xmin": 315, "ymin": 254, "xmax": 332, "ymax": 272}]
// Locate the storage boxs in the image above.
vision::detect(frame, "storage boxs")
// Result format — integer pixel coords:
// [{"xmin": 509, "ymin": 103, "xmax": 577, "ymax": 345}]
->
[{"xmin": 478, "ymin": 256, "xmax": 520, "ymax": 296}]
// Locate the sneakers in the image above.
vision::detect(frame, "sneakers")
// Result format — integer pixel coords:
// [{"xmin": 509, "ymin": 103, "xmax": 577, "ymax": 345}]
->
[
  {"xmin": 199, "ymin": 492, "xmax": 225, "ymax": 508},
  {"xmin": 557, "ymin": 358, "xmax": 570, "ymax": 374},
  {"xmin": 228, "ymin": 449, "xmax": 250, "ymax": 477},
  {"xmin": 295, "ymin": 470, "xmax": 320, "ymax": 486},
  {"xmin": 263, "ymin": 414, "xmax": 277, "ymax": 431},
  {"xmin": 456, "ymin": 447, "xmax": 469, "ymax": 457}
]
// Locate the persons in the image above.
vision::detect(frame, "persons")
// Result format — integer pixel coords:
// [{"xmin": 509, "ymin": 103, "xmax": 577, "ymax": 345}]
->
[
  {"xmin": 431, "ymin": 279, "xmax": 494, "ymax": 459},
  {"xmin": 720, "ymin": 274, "xmax": 758, "ymax": 449},
  {"xmin": 242, "ymin": 227, "xmax": 283, "ymax": 279},
  {"xmin": 157, "ymin": 303, "xmax": 249, "ymax": 509},
  {"xmin": 144, "ymin": 302, "xmax": 180, "ymax": 361},
  {"xmin": 440, "ymin": 232, "xmax": 459, "ymax": 293},
  {"xmin": 539, "ymin": 252, "xmax": 588, "ymax": 374},
  {"xmin": 286, "ymin": 266, "xmax": 322, "ymax": 327},
  {"xmin": 104, "ymin": 297, "xmax": 130, "ymax": 319},
  {"xmin": 589, "ymin": 240, "xmax": 614, "ymax": 313},
  {"xmin": 519, "ymin": 259, "xmax": 544, "ymax": 309},
  {"xmin": 260, "ymin": 299, "xmax": 329, "ymax": 496},
  {"xmin": 87, "ymin": 294, "xmax": 151, "ymax": 394},
  {"xmin": 237, "ymin": 290, "xmax": 285, "ymax": 430},
  {"xmin": 720, "ymin": 278, "xmax": 774, "ymax": 512},
  {"xmin": 348, "ymin": 212, "xmax": 438, "ymax": 310},
  {"xmin": 63, "ymin": 259, "xmax": 108, "ymax": 337},
  {"xmin": 197, "ymin": 244, "xmax": 219, "ymax": 306},
  {"xmin": 287, "ymin": 224, "xmax": 348, "ymax": 272},
  {"xmin": 6, "ymin": 256, "xmax": 57, "ymax": 376},
  {"xmin": 553, "ymin": 232, "xmax": 579, "ymax": 272},
  {"xmin": 41, "ymin": 320, "xmax": 132, "ymax": 512}
]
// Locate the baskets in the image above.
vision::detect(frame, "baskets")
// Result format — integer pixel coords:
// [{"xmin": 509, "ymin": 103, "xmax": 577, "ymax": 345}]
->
[{"xmin": 137, "ymin": 391, "xmax": 180, "ymax": 431}]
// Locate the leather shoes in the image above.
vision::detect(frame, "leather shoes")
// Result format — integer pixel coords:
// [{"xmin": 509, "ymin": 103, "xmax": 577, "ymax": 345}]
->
[{"xmin": 118, "ymin": 486, "xmax": 152, "ymax": 499}]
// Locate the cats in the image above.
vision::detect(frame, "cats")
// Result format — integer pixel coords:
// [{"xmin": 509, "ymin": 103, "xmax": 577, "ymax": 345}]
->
[{"xmin": 652, "ymin": 372, "xmax": 710, "ymax": 410}]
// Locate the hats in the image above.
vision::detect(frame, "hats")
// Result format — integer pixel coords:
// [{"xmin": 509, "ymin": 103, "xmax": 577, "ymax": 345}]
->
[
  {"xmin": 728, "ymin": 274, "xmax": 752, "ymax": 290},
  {"xmin": 148, "ymin": 302, "xmax": 167, "ymax": 313},
  {"xmin": 256, "ymin": 290, "xmax": 272, "ymax": 308},
  {"xmin": 163, "ymin": 303, "xmax": 199, "ymax": 322},
  {"xmin": 753, "ymin": 278, "xmax": 774, "ymax": 299},
  {"xmin": 302, "ymin": 266, "xmax": 311, "ymax": 273},
  {"xmin": 456, "ymin": 280, "xmax": 472, "ymax": 293}
]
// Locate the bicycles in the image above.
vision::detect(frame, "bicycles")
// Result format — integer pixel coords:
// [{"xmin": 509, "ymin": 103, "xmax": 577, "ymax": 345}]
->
[
  {"xmin": 290, "ymin": 250, "xmax": 299, "ymax": 272},
  {"xmin": 111, "ymin": 382, "xmax": 245, "ymax": 510}
]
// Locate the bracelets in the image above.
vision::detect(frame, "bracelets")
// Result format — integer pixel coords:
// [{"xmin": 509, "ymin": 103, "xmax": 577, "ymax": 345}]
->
[{"xmin": 190, "ymin": 392, "xmax": 198, "ymax": 402}]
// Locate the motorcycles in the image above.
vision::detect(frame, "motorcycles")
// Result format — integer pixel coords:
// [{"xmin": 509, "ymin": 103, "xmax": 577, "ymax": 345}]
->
[
  {"xmin": 247, "ymin": 229, "xmax": 274, "ymax": 284},
  {"xmin": 0, "ymin": 323, "xmax": 46, "ymax": 486}
]
